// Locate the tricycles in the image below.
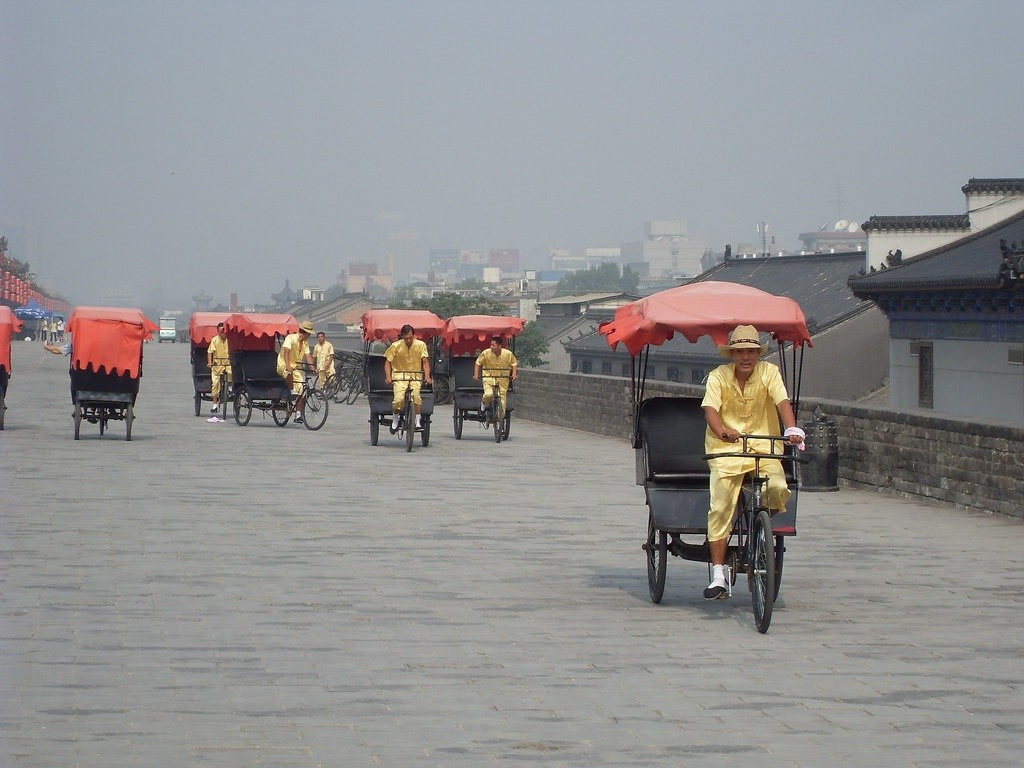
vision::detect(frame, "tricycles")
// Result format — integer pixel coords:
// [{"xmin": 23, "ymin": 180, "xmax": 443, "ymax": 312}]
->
[
  {"xmin": 65, "ymin": 306, "xmax": 161, "ymax": 441},
  {"xmin": 0, "ymin": 305, "xmax": 23, "ymax": 430},
  {"xmin": 441, "ymin": 315, "xmax": 528, "ymax": 443},
  {"xmin": 189, "ymin": 311, "xmax": 329, "ymax": 431},
  {"xmin": 313, "ymin": 350, "xmax": 451, "ymax": 405},
  {"xmin": 360, "ymin": 309, "xmax": 446, "ymax": 452},
  {"xmin": 598, "ymin": 281, "xmax": 813, "ymax": 633}
]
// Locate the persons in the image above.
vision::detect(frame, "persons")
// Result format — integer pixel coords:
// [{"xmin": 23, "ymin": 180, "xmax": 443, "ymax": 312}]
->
[
  {"xmin": 49, "ymin": 319, "xmax": 57, "ymax": 342},
  {"xmin": 383, "ymin": 324, "xmax": 431, "ymax": 435},
  {"xmin": 207, "ymin": 323, "xmax": 234, "ymax": 413},
  {"xmin": 40, "ymin": 317, "xmax": 48, "ymax": 341},
  {"xmin": 312, "ymin": 332, "xmax": 338, "ymax": 400},
  {"xmin": 472, "ymin": 337, "xmax": 519, "ymax": 436},
  {"xmin": 699, "ymin": 324, "xmax": 806, "ymax": 600},
  {"xmin": 56, "ymin": 318, "xmax": 68, "ymax": 342},
  {"xmin": 275, "ymin": 322, "xmax": 318, "ymax": 424},
  {"xmin": 44, "ymin": 340, "xmax": 118, "ymax": 416}
]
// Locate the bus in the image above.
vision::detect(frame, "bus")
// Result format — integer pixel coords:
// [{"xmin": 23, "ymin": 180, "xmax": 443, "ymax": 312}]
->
[{"xmin": 158, "ymin": 317, "xmax": 176, "ymax": 343}]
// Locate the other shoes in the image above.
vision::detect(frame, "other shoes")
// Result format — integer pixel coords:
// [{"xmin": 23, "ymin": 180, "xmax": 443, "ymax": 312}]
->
[
  {"xmin": 389, "ymin": 421, "xmax": 398, "ymax": 435},
  {"xmin": 415, "ymin": 423, "xmax": 422, "ymax": 430},
  {"xmin": 294, "ymin": 417, "xmax": 303, "ymax": 423},
  {"xmin": 501, "ymin": 430, "xmax": 505, "ymax": 437},
  {"xmin": 289, "ymin": 390, "xmax": 300, "ymax": 407},
  {"xmin": 703, "ymin": 586, "xmax": 727, "ymax": 600},
  {"xmin": 210, "ymin": 407, "xmax": 218, "ymax": 414},
  {"xmin": 478, "ymin": 400, "xmax": 487, "ymax": 419}
]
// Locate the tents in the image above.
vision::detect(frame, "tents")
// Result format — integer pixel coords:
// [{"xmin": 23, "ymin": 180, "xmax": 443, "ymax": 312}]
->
[
  {"xmin": 27, "ymin": 297, "xmax": 63, "ymax": 338},
  {"xmin": 13, "ymin": 305, "xmax": 52, "ymax": 341}
]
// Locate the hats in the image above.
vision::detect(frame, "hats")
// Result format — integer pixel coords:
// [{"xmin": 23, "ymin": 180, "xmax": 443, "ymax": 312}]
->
[
  {"xmin": 298, "ymin": 321, "xmax": 315, "ymax": 334},
  {"xmin": 719, "ymin": 324, "xmax": 769, "ymax": 358}
]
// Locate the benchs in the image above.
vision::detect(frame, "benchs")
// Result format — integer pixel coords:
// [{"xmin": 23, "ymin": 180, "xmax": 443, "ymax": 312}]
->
[
  {"xmin": 71, "ymin": 359, "xmax": 140, "ymax": 393},
  {"xmin": 363, "ymin": 356, "xmax": 435, "ymax": 396},
  {"xmin": 191, "ymin": 347, "xmax": 211, "ymax": 376},
  {"xmin": 634, "ymin": 396, "xmax": 798, "ymax": 486},
  {"xmin": 448, "ymin": 356, "xmax": 513, "ymax": 392},
  {"xmin": 230, "ymin": 350, "xmax": 286, "ymax": 382}
]
